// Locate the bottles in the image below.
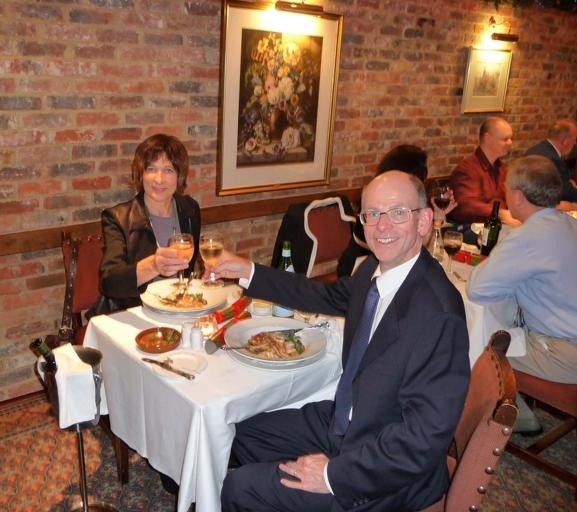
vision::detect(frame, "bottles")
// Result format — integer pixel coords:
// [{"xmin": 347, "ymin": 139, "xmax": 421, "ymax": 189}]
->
[
  {"xmin": 33, "ymin": 336, "xmax": 58, "ymax": 371},
  {"xmin": 271, "ymin": 241, "xmax": 294, "ymax": 318},
  {"xmin": 189, "ymin": 322, "xmax": 202, "ymax": 350},
  {"xmin": 477, "ymin": 200, "xmax": 500, "ymax": 255},
  {"xmin": 180, "ymin": 322, "xmax": 192, "ymax": 346},
  {"xmin": 426, "ymin": 221, "xmax": 444, "ymax": 267}
]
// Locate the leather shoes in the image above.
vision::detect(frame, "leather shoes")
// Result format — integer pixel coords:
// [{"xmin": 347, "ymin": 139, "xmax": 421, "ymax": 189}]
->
[{"xmin": 513, "ymin": 414, "xmax": 542, "ymax": 437}]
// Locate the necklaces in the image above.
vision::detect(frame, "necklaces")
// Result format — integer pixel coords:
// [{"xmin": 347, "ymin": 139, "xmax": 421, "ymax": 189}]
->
[{"xmin": 149, "ymin": 200, "xmax": 178, "ymax": 249}]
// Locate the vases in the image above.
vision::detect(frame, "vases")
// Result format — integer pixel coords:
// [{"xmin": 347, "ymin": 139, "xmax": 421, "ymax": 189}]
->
[{"xmin": 269, "ymin": 108, "xmax": 285, "ymax": 137}]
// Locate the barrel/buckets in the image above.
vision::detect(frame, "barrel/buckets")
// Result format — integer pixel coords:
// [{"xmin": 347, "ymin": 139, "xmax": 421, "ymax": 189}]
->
[{"xmin": 35, "ymin": 345, "xmax": 104, "ymax": 431}]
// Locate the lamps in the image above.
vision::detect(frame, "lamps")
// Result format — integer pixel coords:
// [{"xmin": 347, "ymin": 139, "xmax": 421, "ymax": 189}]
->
[
  {"xmin": 275, "ymin": 0, "xmax": 325, "ymax": 15},
  {"xmin": 491, "ymin": 31, "xmax": 520, "ymax": 42}
]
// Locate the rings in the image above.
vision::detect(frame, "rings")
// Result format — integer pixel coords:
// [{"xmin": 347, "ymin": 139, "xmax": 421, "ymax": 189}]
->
[{"xmin": 291, "ymin": 469, "xmax": 300, "ymax": 476}]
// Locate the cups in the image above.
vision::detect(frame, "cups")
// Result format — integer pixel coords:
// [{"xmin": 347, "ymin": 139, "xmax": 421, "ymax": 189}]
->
[{"xmin": 197, "ymin": 316, "xmax": 219, "ymax": 340}]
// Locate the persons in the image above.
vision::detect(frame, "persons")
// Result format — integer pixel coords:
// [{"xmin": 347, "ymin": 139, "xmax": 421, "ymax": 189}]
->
[
  {"xmin": 204, "ymin": 170, "xmax": 470, "ymax": 510},
  {"xmin": 465, "ymin": 156, "xmax": 577, "ymax": 436},
  {"xmin": 338, "ymin": 145, "xmax": 458, "ymax": 280},
  {"xmin": 85, "ymin": 134, "xmax": 201, "ymax": 324},
  {"xmin": 526, "ymin": 118, "xmax": 577, "ymax": 203},
  {"xmin": 447, "ymin": 116, "xmax": 577, "ymax": 227}
]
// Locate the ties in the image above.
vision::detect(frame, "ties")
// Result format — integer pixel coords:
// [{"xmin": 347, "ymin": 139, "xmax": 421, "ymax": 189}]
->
[{"xmin": 334, "ymin": 277, "xmax": 380, "ymax": 435}]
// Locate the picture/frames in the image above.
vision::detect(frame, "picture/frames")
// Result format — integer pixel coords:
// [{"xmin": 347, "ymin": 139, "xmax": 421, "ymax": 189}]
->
[
  {"xmin": 215, "ymin": 0, "xmax": 343, "ymax": 196},
  {"xmin": 460, "ymin": 47, "xmax": 513, "ymax": 113}
]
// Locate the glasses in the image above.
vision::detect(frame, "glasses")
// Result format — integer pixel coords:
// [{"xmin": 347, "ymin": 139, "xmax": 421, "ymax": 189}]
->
[{"xmin": 358, "ymin": 206, "xmax": 422, "ymax": 226}]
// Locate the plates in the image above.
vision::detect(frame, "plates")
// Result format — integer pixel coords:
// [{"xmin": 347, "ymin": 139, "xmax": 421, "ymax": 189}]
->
[
  {"xmin": 222, "ymin": 316, "xmax": 328, "ymax": 370},
  {"xmin": 139, "ymin": 277, "xmax": 226, "ymax": 314}
]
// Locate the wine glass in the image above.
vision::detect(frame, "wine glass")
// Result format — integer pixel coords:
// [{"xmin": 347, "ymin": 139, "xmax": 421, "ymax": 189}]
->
[
  {"xmin": 169, "ymin": 233, "xmax": 193, "ymax": 297},
  {"xmin": 442, "ymin": 230, "xmax": 463, "ymax": 279},
  {"xmin": 197, "ymin": 236, "xmax": 227, "ymax": 290},
  {"xmin": 432, "ymin": 185, "xmax": 455, "ymax": 229}
]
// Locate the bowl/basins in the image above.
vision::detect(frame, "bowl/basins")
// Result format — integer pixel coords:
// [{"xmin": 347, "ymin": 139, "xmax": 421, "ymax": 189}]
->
[{"xmin": 136, "ymin": 325, "xmax": 181, "ymax": 354}]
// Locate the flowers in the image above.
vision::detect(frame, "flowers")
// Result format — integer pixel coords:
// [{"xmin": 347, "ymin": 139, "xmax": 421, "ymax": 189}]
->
[{"xmin": 241, "ymin": 30, "xmax": 317, "ymax": 158}]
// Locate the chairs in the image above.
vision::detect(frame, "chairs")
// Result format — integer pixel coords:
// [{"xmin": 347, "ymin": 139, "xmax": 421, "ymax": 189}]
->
[
  {"xmin": 507, "ymin": 367, "xmax": 577, "ymax": 485},
  {"xmin": 60, "ymin": 231, "xmax": 129, "ymax": 483},
  {"xmin": 417, "ymin": 330, "xmax": 519, "ymax": 512},
  {"xmin": 426, "ymin": 175, "xmax": 452, "ymax": 219},
  {"xmin": 290, "ymin": 197, "xmax": 357, "ymax": 284}
]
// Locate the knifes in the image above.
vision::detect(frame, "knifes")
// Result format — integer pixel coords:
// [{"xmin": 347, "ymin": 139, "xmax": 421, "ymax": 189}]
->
[
  {"xmin": 257, "ymin": 322, "xmax": 329, "ymax": 338},
  {"xmin": 142, "ymin": 356, "xmax": 194, "ymax": 380}
]
